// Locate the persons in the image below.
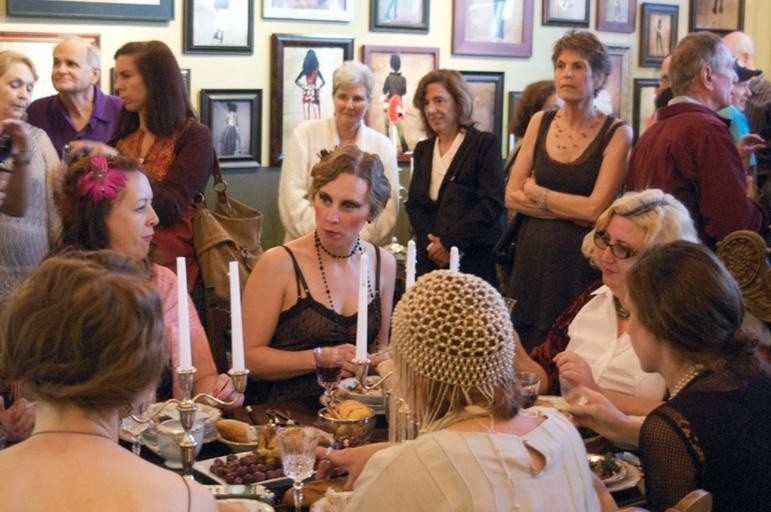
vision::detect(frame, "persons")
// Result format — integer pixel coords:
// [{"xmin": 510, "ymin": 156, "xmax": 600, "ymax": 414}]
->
[
  {"xmin": 241, "ymin": 60, "xmax": 400, "ymax": 404},
  {"xmin": 405, "ymin": 31, "xmax": 771, "ymax": 450},
  {"xmin": 655, "ymin": 18, "xmax": 665, "ymax": 52},
  {"xmin": 0, "ymin": 244, "xmax": 221, "ymax": 512},
  {"xmin": 565, "ymin": 238, "xmax": 771, "ymax": 511},
  {"xmin": 0, "ymin": 34, "xmax": 245, "ymax": 437},
  {"xmin": 309, "ymin": 268, "xmax": 620, "ymax": 511}
]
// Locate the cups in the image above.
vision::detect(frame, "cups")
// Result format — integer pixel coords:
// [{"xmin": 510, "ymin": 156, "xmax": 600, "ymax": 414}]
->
[
  {"xmin": 159, "ymin": 420, "xmax": 207, "ymax": 467},
  {"xmin": 512, "ymin": 370, "xmax": 542, "ymax": 408},
  {"xmin": 559, "ymin": 373, "xmax": 586, "ymax": 405}
]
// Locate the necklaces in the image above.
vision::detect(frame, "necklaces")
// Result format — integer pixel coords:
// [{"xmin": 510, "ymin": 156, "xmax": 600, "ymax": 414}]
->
[{"xmin": 666, "ymin": 363, "xmax": 705, "ymax": 402}]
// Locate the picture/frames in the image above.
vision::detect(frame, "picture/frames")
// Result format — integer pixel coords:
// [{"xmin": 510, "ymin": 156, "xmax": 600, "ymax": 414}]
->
[
  {"xmin": 639, "ymin": 2, "xmax": 679, "ymax": 68},
  {"xmin": 457, "ymin": 70, "xmax": 505, "ymax": 159},
  {"xmin": 595, "ymin": 0, "xmax": 636, "ymax": 33},
  {"xmin": 199, "ymin": 89, "xmax": 263, "ymax": 172},
  {"xmin": 109, "ymin": 68, "xmax": 190, "ymax": 103},
  {"xmin": 689, "ymin": 0, "xmax": 746, "ymax": 36},
  {"xmin": 362, "ymin": 44, "xmax": 440, "ymax": 164},
  {"xmin": 182, "ymin": 0, "xmax": 254, "ymax": 55},
  {"xmin": 270, "ymin": 34, "xmax": 354, "ymax": 168},
  {"xmin": 6, "ymin": 0, "xmax": 176, "ymax": 24},
  {"xmin": 368, "ymin": 0, "xmax": 430, "ymax": 35},
  {"xmin": 633, "ymin": 79, "xmax": 661, "ymax": 144},
  {"xmin": 507, "ymin": 92, "xmax": 525, "ymax": 161},
  {"xmin": 541, "ymin": 0, "xmax": 591, "ymax": 29},
  {"xmin": 594, "ymin": 43, "xmax": 633, "ymax": 130},
  {"xmin": 261, "ymin": 0, "xmax": 351, "ymax": 23},
  {"xmin": 452, "ymin": 0, "xmax": 533, "ymax": 58},
  {"xmin": 0, "ymin": 34, "xmax": 103, "ymax": 102}
]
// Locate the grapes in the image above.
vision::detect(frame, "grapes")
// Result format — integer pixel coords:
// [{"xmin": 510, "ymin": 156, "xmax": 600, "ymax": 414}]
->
[
  {"xmin": 210, "ymin": 453, "xmax": 283, "ymax": 485},
  {"xmin": 319, "ymin": 435, "xmax": 364, "ymax": 450}
]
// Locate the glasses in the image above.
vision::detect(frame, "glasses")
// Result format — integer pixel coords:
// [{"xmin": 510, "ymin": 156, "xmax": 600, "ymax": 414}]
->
[{"xmin": 593, "ymin": 230, "xmax": 636, "ymax": 259}]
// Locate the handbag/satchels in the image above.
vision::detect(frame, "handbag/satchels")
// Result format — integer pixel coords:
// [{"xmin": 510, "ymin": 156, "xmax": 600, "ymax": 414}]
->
[
  {"xmin": 434, "ymin": 182, "xmax": 506, "ymax": 245},
  {"xmin": 490, "ymin": 212, "xmax": 522, "ymax": 265},
  {"xmin": 199, "ymin": 197, "xmax": 264, "ymax": 313}
]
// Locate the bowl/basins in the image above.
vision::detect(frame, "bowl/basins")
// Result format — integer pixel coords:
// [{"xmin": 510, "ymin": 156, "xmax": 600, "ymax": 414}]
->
[
  {"xmin": 149, "ymin": 398, "xmax": 221, "ymax": 435},
  {"xmin": 338, "ymin": 376, "xmax": 385, "ymax": 404},
  {"xmin": 316, "ymin": 406, "xmax": 379, "ymax": 444}
]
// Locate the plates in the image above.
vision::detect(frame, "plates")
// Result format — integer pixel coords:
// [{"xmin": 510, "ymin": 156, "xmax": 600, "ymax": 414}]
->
[
  {"xmin": 586, "ymin": 452, "xmax": 646, "ymax": 493},
  {"xmin": 119, "ymin": 413, "xmax": 222, "ymax": 446},
  {"xmin": 194, "ymin": 447, "xmax": 318, "ymax": 485},
  {"xmin": 331, "ymin": 393, "xmax": 386, "ymax": 417},
  {"xmin": 216, "ymin": 497, "xmax": 276, "ymax": 512},
  {"xmin": 536, "ymin": 394, "xmax": 573, "ymax": 421},
  {"xmin": 221, "ymin": 427, "xmax": 270, "ymax": 453}
]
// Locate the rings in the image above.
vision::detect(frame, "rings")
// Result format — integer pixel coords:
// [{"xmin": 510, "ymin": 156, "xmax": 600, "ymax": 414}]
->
[{"xmin": 323, "ymin": 447, "xmax": 333, "ymax": 456}]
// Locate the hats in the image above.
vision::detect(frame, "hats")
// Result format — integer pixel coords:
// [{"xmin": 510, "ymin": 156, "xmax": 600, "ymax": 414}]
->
[{"xmin": 733, "ymin": 55, "xmax": 762, "ymax": 81}]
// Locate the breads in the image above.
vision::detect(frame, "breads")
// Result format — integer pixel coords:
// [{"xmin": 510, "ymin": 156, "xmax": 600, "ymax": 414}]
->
[{"xmin": 216, "ymin": 419, "xmax": 258, "ymax": 443}]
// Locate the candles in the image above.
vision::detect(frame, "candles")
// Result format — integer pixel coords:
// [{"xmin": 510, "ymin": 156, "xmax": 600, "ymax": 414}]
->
[
  {"xmin": 450, "ymin": 245, "xmax": 461, "ymax": 271},
  {"xmin": 177, "ymin": 256, "xmax": 191, "ymax": 371},
  {"xmin": 355, "ymin": 254, "xmax": 368, "ymax": 362},
  {"xmin": 229, "ymin": 260, "xmax": 245, "ymax": 372},
  {"xmin": 405, "ymin": 239, "xmax": 415, "ymax": 290}
]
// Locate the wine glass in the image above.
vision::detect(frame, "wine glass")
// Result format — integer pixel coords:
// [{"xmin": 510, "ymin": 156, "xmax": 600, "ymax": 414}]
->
[
  {"xmin": 276, "ymin": 423, "xmax": 319, "ymax": 512},
  {"xmin": 312, "ymin": 348, "xmax": 346, "ymax": 404}
]
